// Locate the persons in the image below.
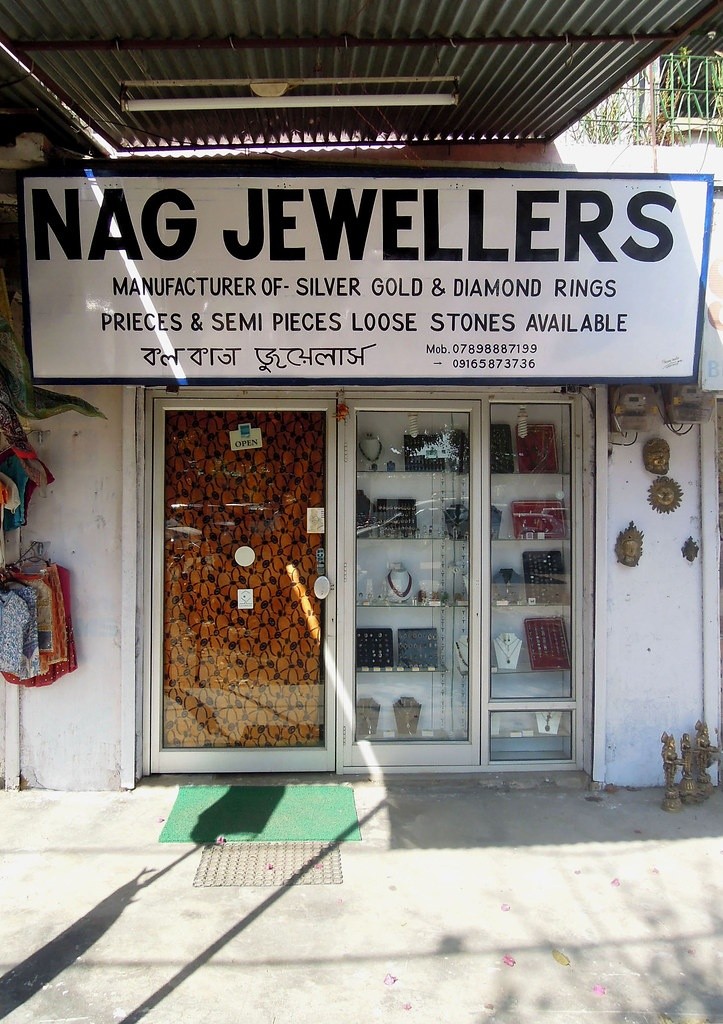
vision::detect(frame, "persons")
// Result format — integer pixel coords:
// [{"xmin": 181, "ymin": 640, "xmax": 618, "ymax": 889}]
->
[
  {"xmin": 680, "ymin": 734, "xmax": 696, "ymax": 778},
  {"xmin": 660, "ymin": 732, "xmax": 682, "ymax": 791},
  {"xmin": 693, "ymin": 721, "xmax": 717, "ymax": 784}
]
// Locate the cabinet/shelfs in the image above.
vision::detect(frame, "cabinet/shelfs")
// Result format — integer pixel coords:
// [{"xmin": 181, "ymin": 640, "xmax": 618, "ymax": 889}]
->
[{"xmin": 356, "ymin": 467, "xmax": 571, "ymax": 741}]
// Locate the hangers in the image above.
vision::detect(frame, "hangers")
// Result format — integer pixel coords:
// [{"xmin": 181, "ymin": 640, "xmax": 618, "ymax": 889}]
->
[{"xmin": 0, "ymin": 541, "xmax": 51, "ymax": 603}]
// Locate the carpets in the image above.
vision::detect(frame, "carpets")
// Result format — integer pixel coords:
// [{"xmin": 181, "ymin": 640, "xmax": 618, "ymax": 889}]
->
[
  {"xmin": 158, "ymin": 784, "xmax": 362, "ymax": 841},
  {"xmin": 192, "ymin": 842, "xmax": 343, "ymax": 886}
]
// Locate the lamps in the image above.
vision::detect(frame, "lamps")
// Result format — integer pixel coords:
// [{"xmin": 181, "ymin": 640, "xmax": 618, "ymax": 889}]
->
[{"xmin": 120, "ymin": 75, "xmax": 460, "ymax": 112}]
